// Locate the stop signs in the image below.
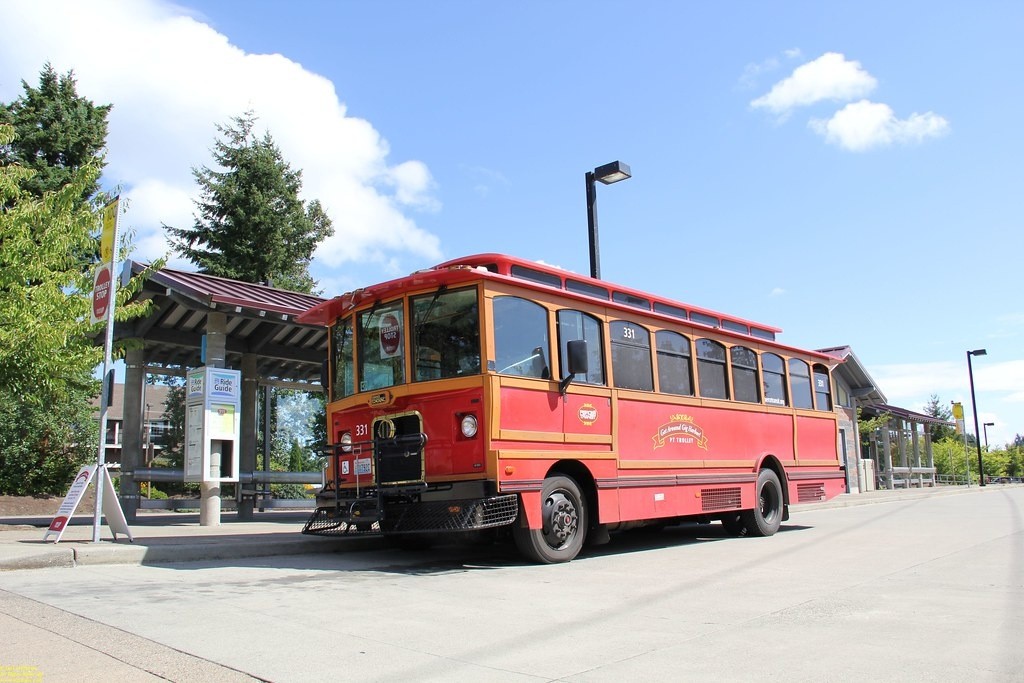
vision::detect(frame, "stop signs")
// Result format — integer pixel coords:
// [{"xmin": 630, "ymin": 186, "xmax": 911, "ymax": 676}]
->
[{"xmin": 91, "ymin": 263, "xmax": 111, "ymax": 325}]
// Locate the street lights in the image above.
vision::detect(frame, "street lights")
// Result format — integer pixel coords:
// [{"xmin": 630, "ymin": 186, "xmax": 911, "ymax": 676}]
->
[
  {"xmin": 982, "ymin": 423, "xmax": 994, "ymax": 452},
  {"xmin": 967, "ymin": 348, "xmax": 988, "ymax": 487},
  {"xmin": 145, "ymin": 404, "xmax": 153, "ymax": 466},
  {"xmin": 585, "ymin": 161, "xmax": 631, "ymax": 279}
]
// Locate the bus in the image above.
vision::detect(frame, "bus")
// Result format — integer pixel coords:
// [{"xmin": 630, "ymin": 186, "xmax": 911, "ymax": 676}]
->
[{"xmin": 294, "ymin": 253, "xmax": 847, "ymax": 564}]
